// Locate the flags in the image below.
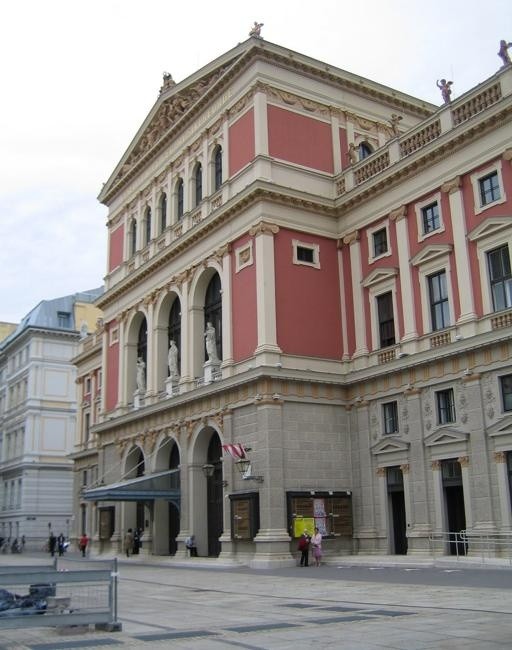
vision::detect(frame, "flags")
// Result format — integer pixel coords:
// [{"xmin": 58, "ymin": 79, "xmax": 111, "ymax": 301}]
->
[{"xmin": 223, "ymin": 442, "xmax": 247, "ymax": 458}]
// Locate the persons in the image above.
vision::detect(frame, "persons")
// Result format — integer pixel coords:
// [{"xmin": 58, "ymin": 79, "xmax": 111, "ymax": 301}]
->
[
  {"xmin": 311, "ymin": 527, "xmax": 322, "ymax": 567},
  {"xmin": 1, "ymin": 533, "xmax": 27, "ymax": 554},
  {"xmin": 168, "ymin": 340, "xmax": 179, "ymax": 376},
  {"xmin": 496, "ymin": 39, "xmax": 512, "ymax": 67},
  {"xmin": 204, "ymin": 322, "xmax": 219, "ymax": 362},
  {"xmin": 124, "ymin": 528, "xmax": 135, "ymax": 557},
  {"xmin": 344, "ymin": 142, "xmax": 360, "ymax": 166},
  {"xmin": 387, "ymin": 114, "xmax": 403, "ymax": 138},
  {"xmin": 134, "ymin": 531, "xmax": 140, "ymax": 554},
  {"xmin": 49, "ymin": 530, "xmax": 57, "ymax": 556},
  {"xmin": 436, "ymin": 79, "xmax": 454, "ymax": 104},
  {"xmin": 185, "ymin": 534, "xmax": 199, "ymax": 556},
  {"xmin": 138, "ymin": 528, "xmax": 144, "ymax": 537},
  {"xmin": 58, "ymin": 533, "xmax": 64, "ymax": 556},
  {"xmin": 297, "ymin": 528, "xmax": 310, "ymax": 567},
  {"xmin": 136, "ymin": 356, "xmax": 146, "ymax": 389},
  {"xmin": 80, "ymin": 533, "xmax": 88, "ymax": 557}
]
